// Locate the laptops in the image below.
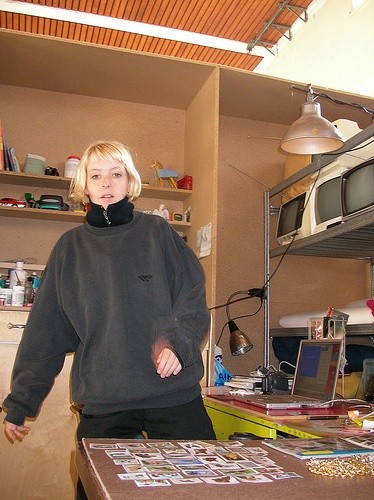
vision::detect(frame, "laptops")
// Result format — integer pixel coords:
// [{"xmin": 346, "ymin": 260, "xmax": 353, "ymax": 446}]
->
[{"xmin": 233, "ymin": 338, "xmax": 344, "ymax": 410}]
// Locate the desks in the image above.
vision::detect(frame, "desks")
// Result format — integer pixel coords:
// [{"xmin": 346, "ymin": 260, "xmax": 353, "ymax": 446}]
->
[{"xmin": 75, "ymin": 435, "xmax": 374, "ymax": 500}]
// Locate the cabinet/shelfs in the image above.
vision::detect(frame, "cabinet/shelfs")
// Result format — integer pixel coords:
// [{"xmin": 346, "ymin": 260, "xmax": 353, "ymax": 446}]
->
[
  {"xmin": 0, "ymin": 171, "xmax": 192, "ymax": 232},
  {"xmin": 263, "ymin": 122, "xmax": 374, "ymax": 392},
  {"xmin": 0, "ymin": 306, "xmax": 76, "ymax": 500}
]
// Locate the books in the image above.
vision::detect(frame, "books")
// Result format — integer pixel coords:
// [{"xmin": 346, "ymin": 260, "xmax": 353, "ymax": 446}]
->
[
  {"xmin": 224, "ymin": 373, "xmax": 294, "ymax": 393},
  {"xmin": 261, "ymin": 432, "xmax": 374, "ymax": 461}
]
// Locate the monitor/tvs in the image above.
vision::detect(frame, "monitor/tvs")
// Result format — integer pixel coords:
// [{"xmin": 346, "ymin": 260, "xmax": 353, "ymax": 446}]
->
[
  {"xmin": 310, "ymin": 166, "xmax": 346, "ymax": 235},
  {"xmin": 276, "ymin": 190, "xmax": 310, "ymax": 246},
  {"xmin": 340, "ymin": 156, "xmax": 374, "ymax": 222}
]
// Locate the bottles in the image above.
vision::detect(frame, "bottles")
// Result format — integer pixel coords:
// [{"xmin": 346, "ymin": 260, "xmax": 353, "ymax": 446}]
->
[
  {"xmin": 9, "ymin": 261, "xmax": 27, "ymax": 288},
  {"xmin": 27, "ymin": 272, "xmax": 41, "ymax": 303},
  {"xmin": 11, "ymin": 285, "xmax": 25, "ymax": 306},
  {"xmin": 64, "ymin": 155, "xmax": 81, "ymax": 179}
]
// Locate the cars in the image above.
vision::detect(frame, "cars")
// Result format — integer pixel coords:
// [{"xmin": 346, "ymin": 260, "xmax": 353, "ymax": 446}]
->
[
  {"xmin": 0, "ymin": 198, "xmax": 27, "ymax": 208},
  {"xmin": 34, "ymin": 195, "xmax": 69, "ymax": 211}
]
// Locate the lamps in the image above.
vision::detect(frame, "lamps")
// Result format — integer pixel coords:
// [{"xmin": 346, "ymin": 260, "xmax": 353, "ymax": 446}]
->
[
  {"xmin": 279, "ymin": 83, "xmax": 345, "ymax": 154},
  {"xmin": 226, "ymin": 287, "xmax": 265, "ymax": 356}
]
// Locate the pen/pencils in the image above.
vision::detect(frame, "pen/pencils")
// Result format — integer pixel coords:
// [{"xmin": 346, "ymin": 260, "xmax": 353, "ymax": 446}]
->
[{"xmin": 306, "ymin": 414, "xmax": 350, "ymax": 420}]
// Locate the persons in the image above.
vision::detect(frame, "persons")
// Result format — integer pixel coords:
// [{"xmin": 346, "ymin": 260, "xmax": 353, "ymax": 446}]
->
[{"xmin": 3, "ymin": 141, "xmax": 217, "ymax": 500}]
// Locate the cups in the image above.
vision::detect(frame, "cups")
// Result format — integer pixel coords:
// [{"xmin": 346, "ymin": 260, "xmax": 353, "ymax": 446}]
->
[{"xmin": 0, "ymin": 289, "xmax": 13, "ymax": 306}]
